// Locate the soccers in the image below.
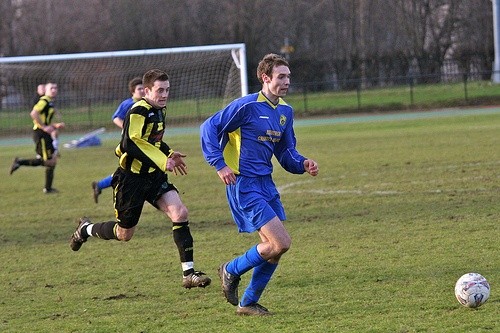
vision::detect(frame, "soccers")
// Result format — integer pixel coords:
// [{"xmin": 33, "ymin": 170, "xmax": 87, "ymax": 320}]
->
[{"xmin": 455, "ymin": 273, "xmax": 491, "ymax": 308}]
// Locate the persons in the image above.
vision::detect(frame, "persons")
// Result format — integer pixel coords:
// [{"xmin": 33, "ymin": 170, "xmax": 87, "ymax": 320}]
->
[
  {"xmin": 10, "ymin": 81, "xmax": 66, "ymax": 195},
  {"xmin": 71, "ymin": 69, "xmax": 211, "ymax": 290},
  {"xmin": 91, "ymin": 77, "xmax": 144, "ymax": 204},
  {"xmin": 32, "ymin": 81, "xmax": 47, "ymax": 109},
  {"xmin": 200, "ymin": 53, "xmax": 319, "ymax": 317}
]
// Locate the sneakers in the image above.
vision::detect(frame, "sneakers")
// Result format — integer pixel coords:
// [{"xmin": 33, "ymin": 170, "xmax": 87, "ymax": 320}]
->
[
  {"xmin": 70, "ymin": 217, "xmax": 93, "ymax": 251},
  {"xmin": 92, "ymin": 182, "xmax": 101, "ymax": 203},
  {"xmin": 237, "ymin": 302, "xmax": 274, "ymax": 316},
  {"xmin": 43, "ymin": 187, "xmax": 58, "ymax": 193},
  {"xmin": 183, "ymin": 271, "xmax": 211, "ymax": 289},
  {"xmin": 218, "ymin": 262, "xmax": 241, "ymax": 306},
  {"xmin": 10, "ymin": 158, "xmax": 19, "ymax": 175}
]
